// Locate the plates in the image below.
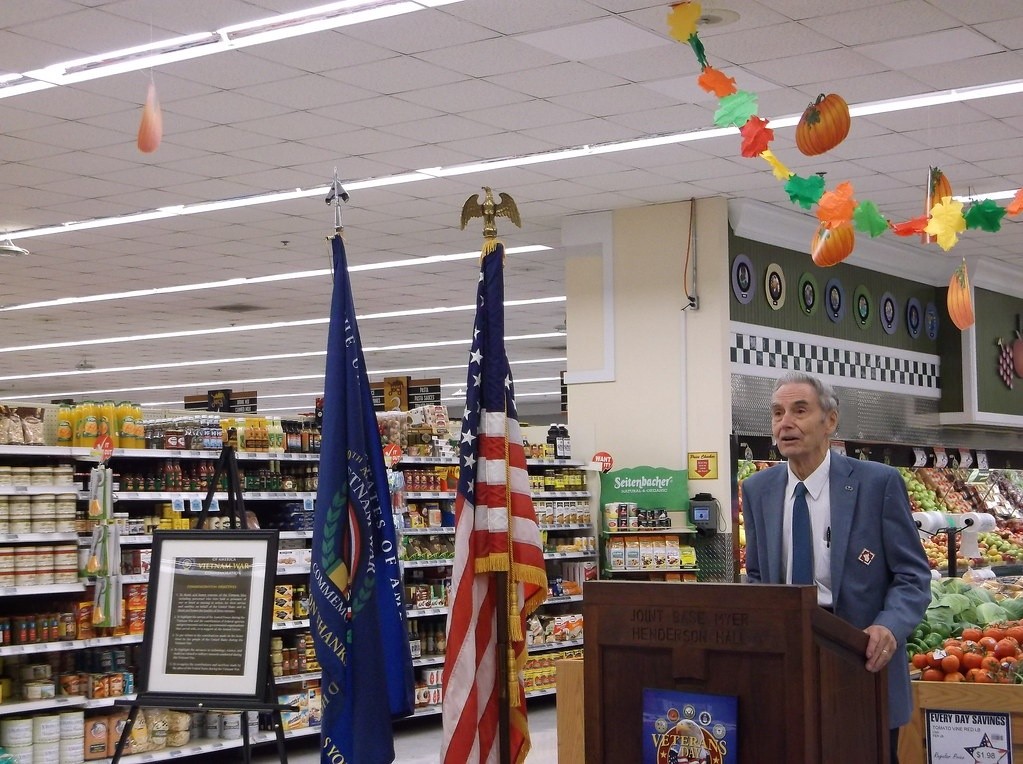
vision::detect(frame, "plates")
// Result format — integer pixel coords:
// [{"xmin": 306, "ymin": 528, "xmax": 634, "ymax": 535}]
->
[
  {"xmin": 122, "ymin": 422, "xmax": 135, "ymax": 434},
  {"xmin": 305, "ymin": 557, "xmax": 311, "ymax": 564},
  {"xmin": 85, "ymin": 422, "xmax": 98, "ymax": 435},
  {"xmin": 278, "ymin": 557, "xmax": 298, "ymax": 566},
  {"xmin": 135, "ymin": 425, "xmax": 144, "ymax": 436},
  {"xmin": 100, "ymin": 422, "xmax": 108, "ymax": 434},
  {"xmin": 57, "ymin": 425, "xmax": 72, "ymax": 439}
]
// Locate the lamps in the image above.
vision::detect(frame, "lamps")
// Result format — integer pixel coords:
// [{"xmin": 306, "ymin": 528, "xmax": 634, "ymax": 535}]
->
[
  {"xmin": 0, "ymin": 78, "xmax": 1023, "ymax": 243},
  {"xmin": 0, "ymin": 0, "xmax": 462, "ymax": 94},
  {"xmin": 0, "ymin": 239, "xmax": 29, "ymax": 257}
]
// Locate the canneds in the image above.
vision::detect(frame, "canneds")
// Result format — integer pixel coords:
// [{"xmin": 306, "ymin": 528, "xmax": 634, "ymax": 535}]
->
[
  {"xmin": 0, "ymin": 612, "xmax": 135, "ymax": 707},
  {"xmin": 549, "ymin": 536, "xmax": 595, "ymax": 550},
  {"xmin": 188, "ymin": 709, "xmax": 259, "ymax": 741},
  {"xmin": 270, "ymin": 633, "xmax": 307, "ymax": 677},
  {"xmin": 402, "ymin": 470, "xmax": 441, "ymax": 492}
]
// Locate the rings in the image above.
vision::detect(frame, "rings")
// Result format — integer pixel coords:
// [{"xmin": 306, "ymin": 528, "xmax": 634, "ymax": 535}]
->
[{"xmin": 883, "ymin": 649, "xmax": 888, "ymax": 654}]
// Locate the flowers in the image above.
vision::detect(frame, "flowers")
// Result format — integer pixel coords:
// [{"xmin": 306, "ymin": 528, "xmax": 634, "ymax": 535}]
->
[{"xmin": 666, "ymin": 0, "xmax": 1023, "ymax": 251}]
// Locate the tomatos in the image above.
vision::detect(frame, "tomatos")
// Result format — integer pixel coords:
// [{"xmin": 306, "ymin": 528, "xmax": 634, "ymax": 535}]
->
[{"xmin": 912, "ymin": 617, "xmax": 1023, "ymax": 683}]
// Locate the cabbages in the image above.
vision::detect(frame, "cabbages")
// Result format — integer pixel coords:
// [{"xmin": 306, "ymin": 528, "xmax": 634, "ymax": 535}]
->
[{"xmin": 926, "ymin": 577, "xmax": 1023, "ymax": 627}]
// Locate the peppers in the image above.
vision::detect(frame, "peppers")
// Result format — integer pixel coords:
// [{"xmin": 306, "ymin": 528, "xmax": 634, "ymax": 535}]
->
[{"xmin": 905, "ymin": 613, "xmax": 964, "ymax": 663}]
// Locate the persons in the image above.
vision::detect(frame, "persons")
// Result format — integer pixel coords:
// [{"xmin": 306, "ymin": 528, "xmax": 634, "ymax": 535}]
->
[{"xmin": 742, "ymin": 371, "xmax": 932, "ymax": 764}]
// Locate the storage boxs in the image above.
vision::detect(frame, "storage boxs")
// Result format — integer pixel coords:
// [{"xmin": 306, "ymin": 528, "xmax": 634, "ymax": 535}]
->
[
  {"xmin": 305, "ymin": 687, "xmax": 322, "ymax": 727},
  {"xmin": 276, "ymin": 681, "xmax": 309, "ymax": 731},
  {"xmin": 275, "ymin": 585, "xmax": 294, "ymax": 598},
  {"xmin": 273, "ymin": 609, "xmax": 294, "ymax": 623},
  {"xmin": 122, "ymin": 550, "xmax": 133, "ymax": 574},
  {"xmin": 273, "ymin": 598, "xmax": 293, "ymax": 609},
  {"xmin": 133, "ymin": 549, "xmax": 152, "ymax": 574},
  {"xmin": 898, "ymin": 669, "xmax": 1023, "ymax": 764},
  {"xmin": 301, "ymin": 680, "xmax": 319, "ymax": 689}
]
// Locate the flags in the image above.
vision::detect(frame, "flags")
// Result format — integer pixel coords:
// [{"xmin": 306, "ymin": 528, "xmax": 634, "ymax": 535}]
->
[
  {"xmin": 440, "ymin": 238, "xmax": 548, "ymax": 764},
  {"xmin": 309, "ymin": 233, "xmax": 415, "ymax": 764}
]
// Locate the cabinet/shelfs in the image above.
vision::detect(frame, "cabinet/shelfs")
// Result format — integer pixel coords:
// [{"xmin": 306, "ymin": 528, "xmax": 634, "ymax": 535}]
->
[{"xmin": 0, "ymin": 435, "xmax": 699, "ymax": 764}]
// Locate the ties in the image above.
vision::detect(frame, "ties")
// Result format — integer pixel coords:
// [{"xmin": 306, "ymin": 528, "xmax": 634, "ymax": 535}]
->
[{"xmin": 792, "ymin": 482, "xmax": 814, "ymax": 585}]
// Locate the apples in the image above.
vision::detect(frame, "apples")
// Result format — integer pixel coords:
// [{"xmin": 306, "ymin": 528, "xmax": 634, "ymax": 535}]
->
[
  {"xmin": 737, "ymin": 460, "xmax": 782, "ymax": 483},
  {"xmin": 897, "ymin": 468, "xmax": 973, "ymax": 513},
  {"xmin": 919, "ymin": 531, "xmax": 1023, "ymax": 570}
]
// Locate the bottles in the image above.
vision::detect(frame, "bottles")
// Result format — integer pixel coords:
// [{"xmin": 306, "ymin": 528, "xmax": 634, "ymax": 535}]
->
[
  {"xmin": 523, "ymin": 437, "xmax": 543, "ymax": 458},
  {"xmin": 245, "ymin": 461, "xmax": 296, "ymax": 491},
  {"xmin": 406, "ymin": 620, "xmax": 426, "ymax": 659},
  {"xmin": 297, "ymin": 462, "xmax": 319, "ymax": 492},
  {"xmin": 246, "ymin": 416, "xmax": 322, "ymax": 453},
  {"xmin": 155, "ymin": 459, "xmax": 190, "ymax": 492},
  {"xmin": 217, "ymin": 463, "xmax": 244, "ymax": 492},
  {"xmin": 293, "ymin": 585, "xmax": 309, "ymax": 620},
  {"xmin": 548, "ymin": 423, "xmax": 571, "ymax": 459},
  {"xmin": 121, "ymin": 472, "xmax": 155, "ymax": 492},
  {"xmin": 189, "ymin": 460, "xmax": 215, "ymax": 491},
  {"xmin": 57, "ymin": 400, "xmax": 245, "ymax": 452},
  {"xmin": 190, "ymin": 512, "xmax": 242, "ymax": 529},
  {"xmin": 427, "ymin": 623, "xmax": 445, "ymax": 655}
]
